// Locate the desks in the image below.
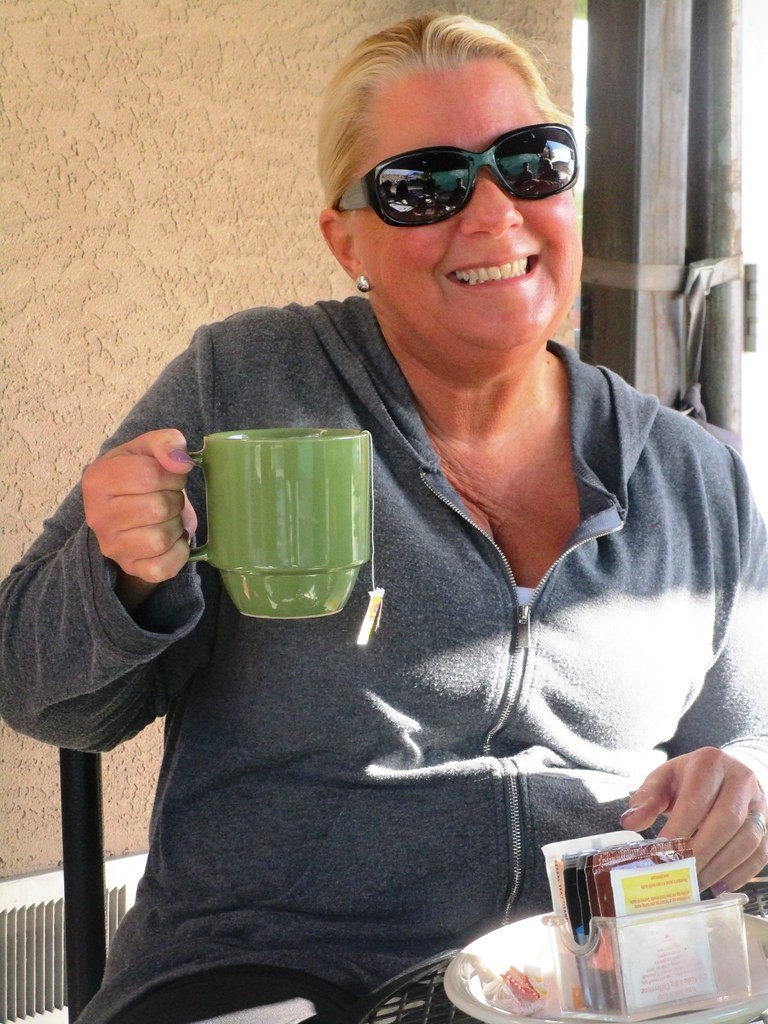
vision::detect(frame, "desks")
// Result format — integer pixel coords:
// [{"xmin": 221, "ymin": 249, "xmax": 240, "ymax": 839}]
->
[{"xmin": 339, "ymin": 881, "xmax": 768, "ymax": 1024}]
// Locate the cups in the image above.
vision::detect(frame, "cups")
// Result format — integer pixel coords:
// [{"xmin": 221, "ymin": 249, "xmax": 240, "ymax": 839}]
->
[{"xmin": 165, "ymin": 428, "xmax": 372, "ymax": 620}]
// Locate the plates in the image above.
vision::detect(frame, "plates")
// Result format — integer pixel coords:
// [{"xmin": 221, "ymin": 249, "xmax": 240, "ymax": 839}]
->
[{"xmin": 442, "ymin": 907, "xmax": 768, "ymax": 1024}]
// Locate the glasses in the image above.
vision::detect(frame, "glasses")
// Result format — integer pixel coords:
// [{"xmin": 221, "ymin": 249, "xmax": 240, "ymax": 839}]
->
[{"xmin": 332, "ymin": 122, "xmax": 580, "ymax": 227}]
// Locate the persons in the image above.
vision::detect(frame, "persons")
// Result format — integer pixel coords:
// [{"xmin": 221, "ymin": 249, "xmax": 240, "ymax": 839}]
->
[{"xmin": 1, "ymin": 12, "xmax": 768, "ymax": 1024}]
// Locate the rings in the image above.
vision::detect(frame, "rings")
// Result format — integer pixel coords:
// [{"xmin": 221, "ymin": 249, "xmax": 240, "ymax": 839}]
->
[{"xmin": 745, "ymin": 811, "xmax": 767, "ymax": 834}]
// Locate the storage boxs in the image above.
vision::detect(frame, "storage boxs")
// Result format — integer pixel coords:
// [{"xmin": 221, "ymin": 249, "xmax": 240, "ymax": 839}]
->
[{"xmin": 541, "ymin": 892, "xmax": 751, "ymax": 1024}]
